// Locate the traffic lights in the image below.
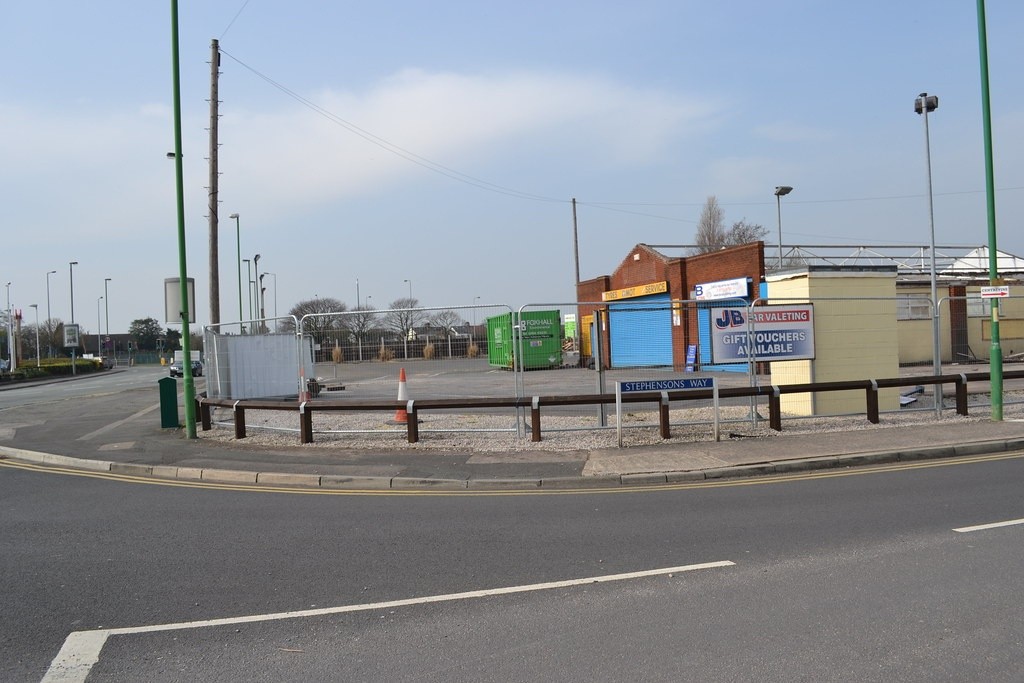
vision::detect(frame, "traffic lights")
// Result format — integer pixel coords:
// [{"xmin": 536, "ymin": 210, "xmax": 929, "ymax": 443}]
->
[
  {"xmin": 128, "ymin": 342, "xmax": 132, "ymax": 351},
  {"xmin": 69, "ymin": 349, "xmax": 72, "ymax": 355}
]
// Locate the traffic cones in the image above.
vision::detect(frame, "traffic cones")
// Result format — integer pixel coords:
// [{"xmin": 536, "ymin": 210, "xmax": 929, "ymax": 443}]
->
[
  {"xmin": 385, "ymin": 367, "xmax": 425, "ymax": 425},
  {"xmin": 297, "ymin": 367, "xmax": 312, "ymax": 403}
]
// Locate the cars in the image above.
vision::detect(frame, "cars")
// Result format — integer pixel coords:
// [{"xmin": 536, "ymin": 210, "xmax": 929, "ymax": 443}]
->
[
  {"xmin": 170, "ymin": 359, "xmax": 202, "ymax": 377},
  {"xmin": 101, "ymin": 355, "xmax": 114, "ymax": 369}
]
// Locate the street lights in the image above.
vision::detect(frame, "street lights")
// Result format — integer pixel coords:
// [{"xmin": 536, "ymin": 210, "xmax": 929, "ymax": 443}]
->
[
  {"xmin": 474, "ymin": 297, "xmax": 481, "ymax": 345},
  {"xmin": 29, "ymin": 304, "xmax": 40, "ymax": 366},
  {"xmin": 69, "ymin": 262, "xmax": 77, "ymax": 376},
  {"xmin": 263, "ymin": 272, "xmax": 277, "ymax": 334},
  {"xmin": 243, "ymin": 259, "xmax": 252, "ymax": 334},
  {"xmin": 404, "ymin": 279, "xmax": 414, "ymax": 329},
  {"xmin": 259, "ymin": 274, "xmax": 265, "ymax": 333},
  {"xmin": 254, "ymin": 254, "xmax": 259, "ymax": 335},
  {"xmin": 105, "ymin": 278, "xmax": 111, "ymax": 360},
  {"xmin": 228, "ymin": 213, "xmax": 243, "ymax": 335},
  {"xmin": 914, "ymin": 93, "xmax": 949, "ymax": 411},
  {"xmin": 366, "ymin": 295, "xmax": 371, "ymax": 310},
  {"xmin": 250, "ymin": 281, "xmax": 258, "ymax": 335},
  {"xmin": 46, "ymin": 271, "xmax": 57, "ymax": 358},
  {"xmin": 98, "ymin": 297, "xmax": 103, "ymax": 357},
  {"xmin": 4, "ymin": 282, "xmax": 11, "ymax": 360},
  {"xmin": 774, "ymin": 185, "xmax": 794, "ymax": 270}
]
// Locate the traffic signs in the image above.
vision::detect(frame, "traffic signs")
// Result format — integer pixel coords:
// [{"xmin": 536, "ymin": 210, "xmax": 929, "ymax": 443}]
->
[{"xmin": 980, "ymin": 286, "xmax": 1010, "ymax": 298}]
